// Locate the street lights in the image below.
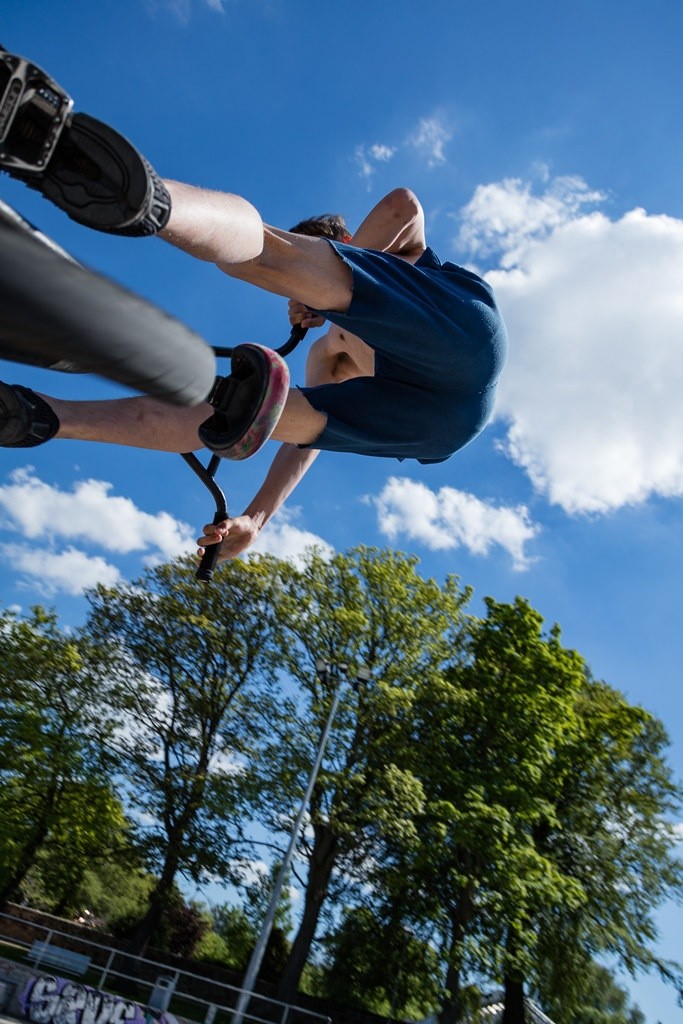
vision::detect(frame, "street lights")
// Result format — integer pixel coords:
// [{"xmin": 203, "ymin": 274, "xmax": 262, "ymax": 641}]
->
[{"xmin": 231, "ymin": 659, "xmax": 372, "ymax": 1024}]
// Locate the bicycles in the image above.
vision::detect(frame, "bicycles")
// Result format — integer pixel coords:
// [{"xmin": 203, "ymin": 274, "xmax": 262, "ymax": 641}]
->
[{"xmin": 0, "ymin": 199, "xmax": 309, "ymax": 583}]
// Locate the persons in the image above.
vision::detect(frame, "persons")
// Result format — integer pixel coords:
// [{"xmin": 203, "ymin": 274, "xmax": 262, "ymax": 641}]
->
[{"xmin": 0, "ymin": 47, "xmax": 508, "ymax": 561}]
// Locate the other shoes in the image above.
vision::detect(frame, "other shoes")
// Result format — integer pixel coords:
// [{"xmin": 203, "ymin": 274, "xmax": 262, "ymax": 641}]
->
[
  {"xmin": 0, "ymin": 381, "xmax": 59, "ymax": 449},
  {"xmin": 0, "ymin": 46, "xmax": 171, "ymax": 237}
]
